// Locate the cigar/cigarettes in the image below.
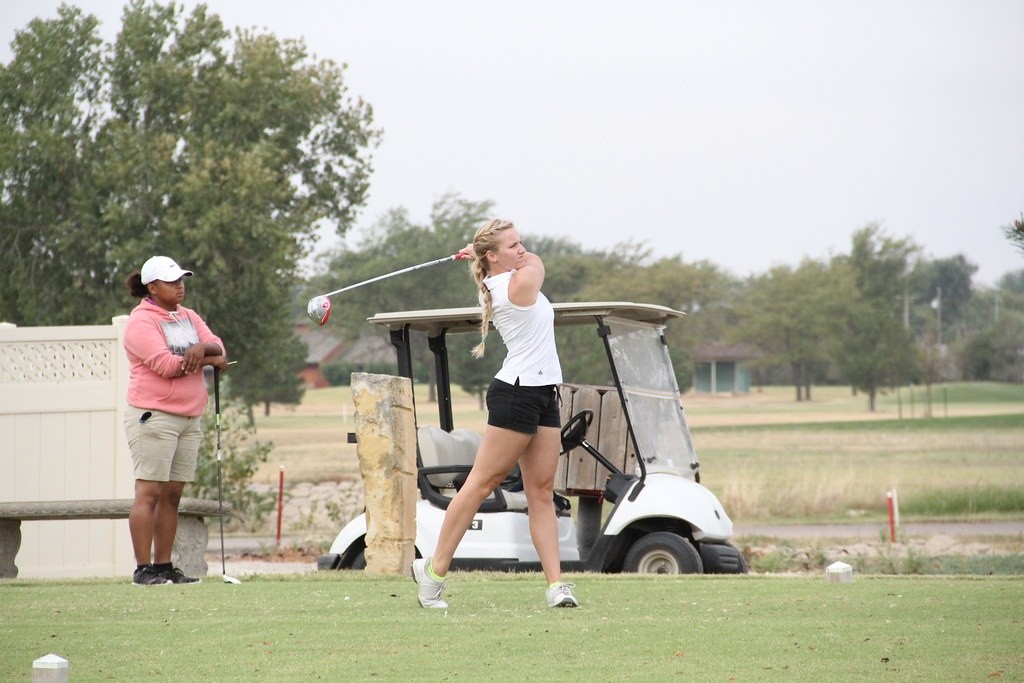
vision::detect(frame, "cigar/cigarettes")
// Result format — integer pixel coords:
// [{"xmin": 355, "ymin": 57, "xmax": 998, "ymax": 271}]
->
[{"xmin": 227, "ymin": 360, "xmax": 238, "ymax": 365}]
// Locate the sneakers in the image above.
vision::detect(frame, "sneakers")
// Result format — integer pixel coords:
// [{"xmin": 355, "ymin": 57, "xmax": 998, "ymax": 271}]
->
[
  {"xmin": 411, "ymin": 557, "xmax": 448, "ymax": 609},
  {"xmin": 158, "ymin": 568, "xmax": 201, "ymax": 584},
  {"xmin": 131, "ymin": 564, "xmax": 173, "ymax": 585},
  {"xmin": 546, "ymin": 582, "xmax": 579, "ymax": 607}
]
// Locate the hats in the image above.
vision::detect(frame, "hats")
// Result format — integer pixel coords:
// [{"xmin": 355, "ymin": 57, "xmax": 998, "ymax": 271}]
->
[{"xmin": 141, "ymin": 256, "xmax": 194, "ymax": 285}]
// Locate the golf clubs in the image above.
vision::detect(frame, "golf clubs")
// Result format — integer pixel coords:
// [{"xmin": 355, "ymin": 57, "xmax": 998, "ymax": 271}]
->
[
  {"xmin": 213, "ymin": 365, "xmax": 242, "ymax": 585},
  {"xmin": 307, "ymin": 252, "xmax": 468, "ymax": 327}
]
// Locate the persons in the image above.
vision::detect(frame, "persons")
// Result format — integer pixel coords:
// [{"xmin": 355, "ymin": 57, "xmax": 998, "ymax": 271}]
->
[
  {"xmin": 121, "ymin": 255, "xmax": 229, "ymax": 586},
  {"xmin": 410, "ymin": 224, "xmax": 579, "ymax": 609}
]
